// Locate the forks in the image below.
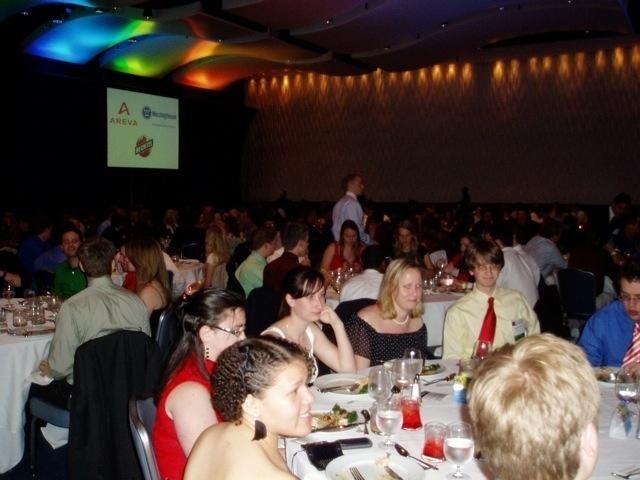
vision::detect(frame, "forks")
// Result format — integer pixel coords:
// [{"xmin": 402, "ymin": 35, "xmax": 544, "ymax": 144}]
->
[
  {"xmin": 349, "ymin": 465, "xmax": 365, "ymax": 480},
  {"xmin": 317, "ymin": 383, "xmax": 360, "ymax": 394}
]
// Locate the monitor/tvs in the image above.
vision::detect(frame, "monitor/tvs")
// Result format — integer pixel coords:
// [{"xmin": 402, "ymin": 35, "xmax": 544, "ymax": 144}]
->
[{"xmin": 106, "ymin": 85, "xmax": 180, "ymax": 170}]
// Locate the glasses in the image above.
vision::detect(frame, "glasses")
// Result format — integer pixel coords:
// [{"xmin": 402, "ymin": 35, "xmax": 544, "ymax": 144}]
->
[{"xmin": 210, "ymin": 325, "xmax": 246, "ymax": 337}]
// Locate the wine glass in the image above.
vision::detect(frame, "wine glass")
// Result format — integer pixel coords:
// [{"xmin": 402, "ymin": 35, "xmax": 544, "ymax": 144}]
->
[
  {"xmin": 423, "ymin": 259, "xmax": 454, "ymax": 295},
  {"xmin": 472, "ymin": 337, "xmax": 491, "ymax": 363},
  {"xmin": 402, "ymin": 347, "xmax": 424, "ymax": 375},
  {"xmin": 3, "ymin": 282, "xmax": 15, "ymax": 309},
  {"xmin": 375, "ymin": 401, "xmax": 404, "ymax": 450},
  {"xmin": 391, "ymin": 359, "xmax": 413, "ymax": 399},
  {"xmin": 369, "ymin": 367, "xmax": 394, "ymax": 401},
  {"xmin": 615, "ymin": 369, "xmax": 639, "ymax": 408},
  {"xmin": 327, "ymin": 262, "xmax": 353, "ymax": 298},
  {"xmin": 442, "ymin": 420, "xmax": 474, "ymax": 480}
]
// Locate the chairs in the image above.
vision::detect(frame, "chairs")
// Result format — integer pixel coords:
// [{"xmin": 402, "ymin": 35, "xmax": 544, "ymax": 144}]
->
[
  {"xmin": 155, "ymin": 302, "xmax": 175, "ymax": 352},
  {"xmin": 574, "ymin": 267, "xmax": 604, "ymax": 299},
  {"xmin": 129, "ymin": 398, "xmax": 162, "ymax": 480},
  {"xmin": 552, "ymin": 267, "xmax": 593, "ymax": 332}
]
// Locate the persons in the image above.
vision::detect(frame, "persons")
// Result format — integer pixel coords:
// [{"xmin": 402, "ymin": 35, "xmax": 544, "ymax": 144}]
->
[
  {"xmin": 463, "ymin": 332, "xmax": 601, "ymax": 480},
  {"xmin": 183, "ymin": 334, "xmax": 316, "ymax": 480},
  {"xmin": 26, "ymin": 236, "xmax": 155, "ymax": 429},
  {"xmin": 1, "ymin": 171, "xmax": 639, "ymax": 379},
  {"xmin": 151, "ymin": 284, "xmax": 252, "ymax": 480}
]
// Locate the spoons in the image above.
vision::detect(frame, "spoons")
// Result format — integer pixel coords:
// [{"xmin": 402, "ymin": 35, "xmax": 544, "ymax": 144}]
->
[
  {"xmin": 362, "ymin": 407, "xmax": 372, "ymax": 437},
  {"xmin": 424, "ymin": 372, "xmax": 456, "ymax": 387},
  {"xmin": 606, "ymin": 467, "xmax": 640, "ymax": 480},
  {"xmin": 393, "ymin": 442, "xmax": 439, "ymax": 472},
  {"xmin": 314, "ymin": 381, "xmax": 385, "ymax": 395}
]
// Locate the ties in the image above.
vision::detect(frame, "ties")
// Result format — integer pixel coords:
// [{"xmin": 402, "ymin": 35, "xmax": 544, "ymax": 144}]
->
[
  {"xmin": 476, "ymin": 298, "xmax": 496, "ymax": 357},
  {"xmin": 621, "ymin": 322, "xmax": 640, "ymax": 368}
]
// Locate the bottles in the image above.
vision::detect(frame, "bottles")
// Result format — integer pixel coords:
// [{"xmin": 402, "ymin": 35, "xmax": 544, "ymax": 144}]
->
[{"xmin": 411, "ymin": 373, "xmax": 423, "ymax": 403}]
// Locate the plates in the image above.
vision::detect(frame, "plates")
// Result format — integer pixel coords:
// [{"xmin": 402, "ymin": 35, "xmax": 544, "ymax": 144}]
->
[
  {"xmin": 594, "ymin": 370, "xmax": 640, "ymax": 386},
  {"xmin": 421, "ymin": 362, "xmax": 447, "ymax": 377},
  {"xmin": 312, "ymin": 372, "xmax": 373, "ymax": 397},
  {"xmin": 323, "ymin": 450, "xmax": 427, "ymax": 480},
  {"xmin": 311, "ymin": 407, "xmax": 358, "ymax": 434}
]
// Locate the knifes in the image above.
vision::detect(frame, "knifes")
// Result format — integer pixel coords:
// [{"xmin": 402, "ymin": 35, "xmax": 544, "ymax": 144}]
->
[{"xmin": 383, "ymin": 464, "xmax": 405, "ymax": 480}]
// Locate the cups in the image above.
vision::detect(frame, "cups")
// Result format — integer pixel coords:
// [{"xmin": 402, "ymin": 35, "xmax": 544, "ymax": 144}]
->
[
  {"xmin": 171, "ymin": 255, "xmax": 179, "ymax": 266},
  {"xmin": 421, "ymin": 421, "xmax": 449, "ymax": 463},
  {"xmin": 400, "ymin": 396, "xmax": 423, "ymax": 431},
  {"xmin": 13, "ymin": 289, "xmax": 59, "ymax": 330},
  {"xmin": 0, "ymin": 307, "xmax": 8, "ymax": 332}
]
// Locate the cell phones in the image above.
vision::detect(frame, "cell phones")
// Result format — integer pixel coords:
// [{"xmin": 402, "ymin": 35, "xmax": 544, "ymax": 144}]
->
[{"xmin": 336, "ymin": 438, "xmax": 372, "ymax": 450}]
